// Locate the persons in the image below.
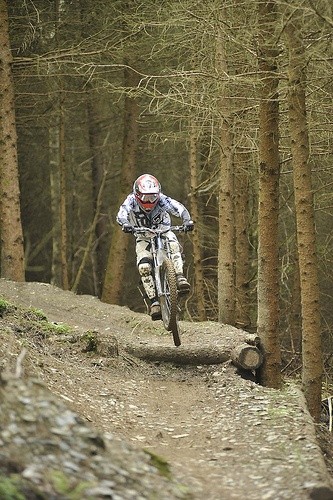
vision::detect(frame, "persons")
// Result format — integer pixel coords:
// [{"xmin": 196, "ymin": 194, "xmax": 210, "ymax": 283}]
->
[{"xmin": 116, "ymin": 173, "xmax": 195, "ymax": 321}]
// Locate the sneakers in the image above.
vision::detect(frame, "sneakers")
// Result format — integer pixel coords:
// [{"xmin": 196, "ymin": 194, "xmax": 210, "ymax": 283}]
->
[
  {"xmin": 178, "ymin": 278, "xmax": 191, "ymax": 298},
  {"xmin": 150, "ymin": 301, "xmax": 162, "ymax": 321}
]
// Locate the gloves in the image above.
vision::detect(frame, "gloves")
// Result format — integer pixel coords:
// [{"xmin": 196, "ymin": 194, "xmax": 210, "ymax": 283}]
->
[
  {"xmin": 122, "ymin": 224, "xmax": 133, "ymax": 233},
  {"xmin": 183, "ymin": 221, "xmax": 194, "ymax": 234}
]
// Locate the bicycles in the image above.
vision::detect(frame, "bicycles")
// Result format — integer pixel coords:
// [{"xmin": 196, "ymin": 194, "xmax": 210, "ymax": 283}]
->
[{"xmin": 126, "ymin": 224, "xmax": 193, "ymax": 347}]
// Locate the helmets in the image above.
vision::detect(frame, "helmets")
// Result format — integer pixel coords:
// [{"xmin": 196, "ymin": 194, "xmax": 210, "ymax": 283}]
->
[{"xmin": 135, "ymin": 174, "xmax": 161, "ymax": 215}]
